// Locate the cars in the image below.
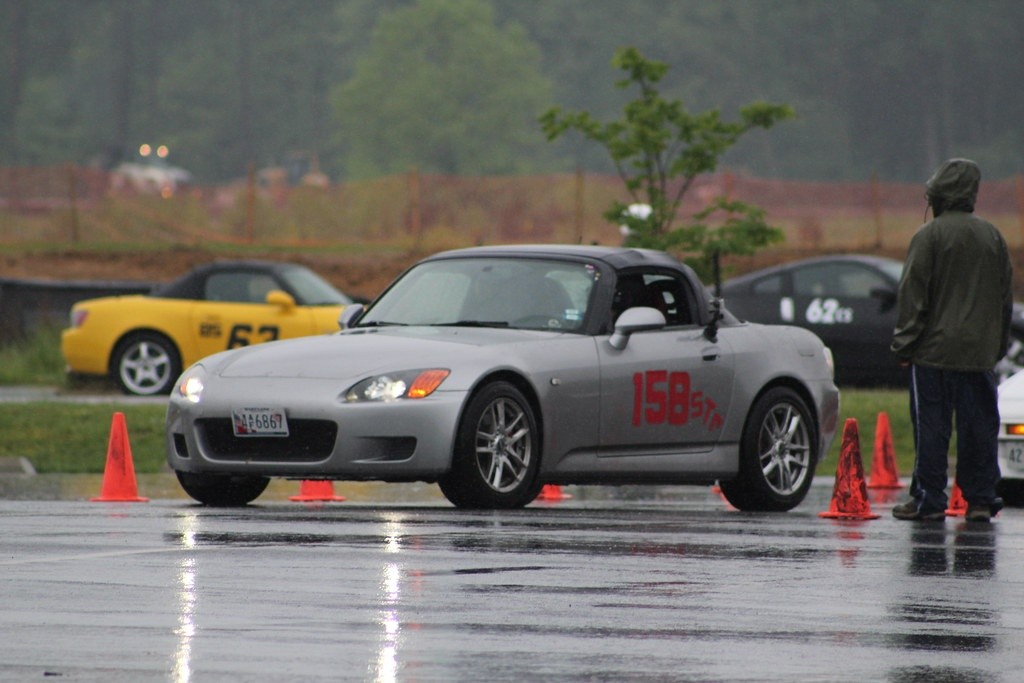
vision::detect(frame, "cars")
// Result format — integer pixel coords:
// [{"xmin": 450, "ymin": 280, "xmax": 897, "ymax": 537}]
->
[{"xmin": 994, "ymin": 366, "xmax": 1024, "ymax": 507}]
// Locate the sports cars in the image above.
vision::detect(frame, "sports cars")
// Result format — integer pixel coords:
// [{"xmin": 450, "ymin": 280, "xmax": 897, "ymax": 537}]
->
[
  {"xmin": 702, "ymin": 252, "xmax": 1024, "ymax": 386},
  {"xmin": 164, "ymin": 243, "xmax": 841, "ymax": 512},
  {"xmin": 60, "ymin": 256, "xmax": 371, "ymax": 397}
]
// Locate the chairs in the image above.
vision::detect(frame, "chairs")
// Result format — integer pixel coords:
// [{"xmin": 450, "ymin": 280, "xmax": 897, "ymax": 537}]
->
[{"xmin": 486, "ymin": 278, "xmax": 574, "ymax": 324}]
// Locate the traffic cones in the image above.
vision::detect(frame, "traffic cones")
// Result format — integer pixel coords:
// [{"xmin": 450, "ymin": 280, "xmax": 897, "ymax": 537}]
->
[
  {"xmin": 819, "ymin": 417, "xmax": 882, "ymax": 520},
  {"xmin": 537, "ymin": 482, "xmax": 572, "ymax": 500},
  {"xmin": 288, "ymin": 479, "xmax": 345, "ymax": 502},
  {"xmin": 943, "ymin": 461, "xmax": 1001, "ymax": 517},
  {"xmin": 85, "ymin": 410, "xmax": 151, "ymax": 503},
  {"xmin": 866, "ymin": 411, "xmax": 905, "ymax": 488}
]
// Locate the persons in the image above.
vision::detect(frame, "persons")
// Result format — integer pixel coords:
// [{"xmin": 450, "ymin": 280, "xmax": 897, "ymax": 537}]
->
[{"xmin": 889, "ymin": 156, "xmax": 1013, "ymax": 520}]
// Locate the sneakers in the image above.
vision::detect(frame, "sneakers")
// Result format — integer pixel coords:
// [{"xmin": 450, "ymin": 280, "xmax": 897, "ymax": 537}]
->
[
  {"xmin": 892, "ymin": 499, "xmax": 945, "ymax": 521},
  {"xmin": 964, "ymin": 504, "xmax": 991, "ymax": 522}
]
